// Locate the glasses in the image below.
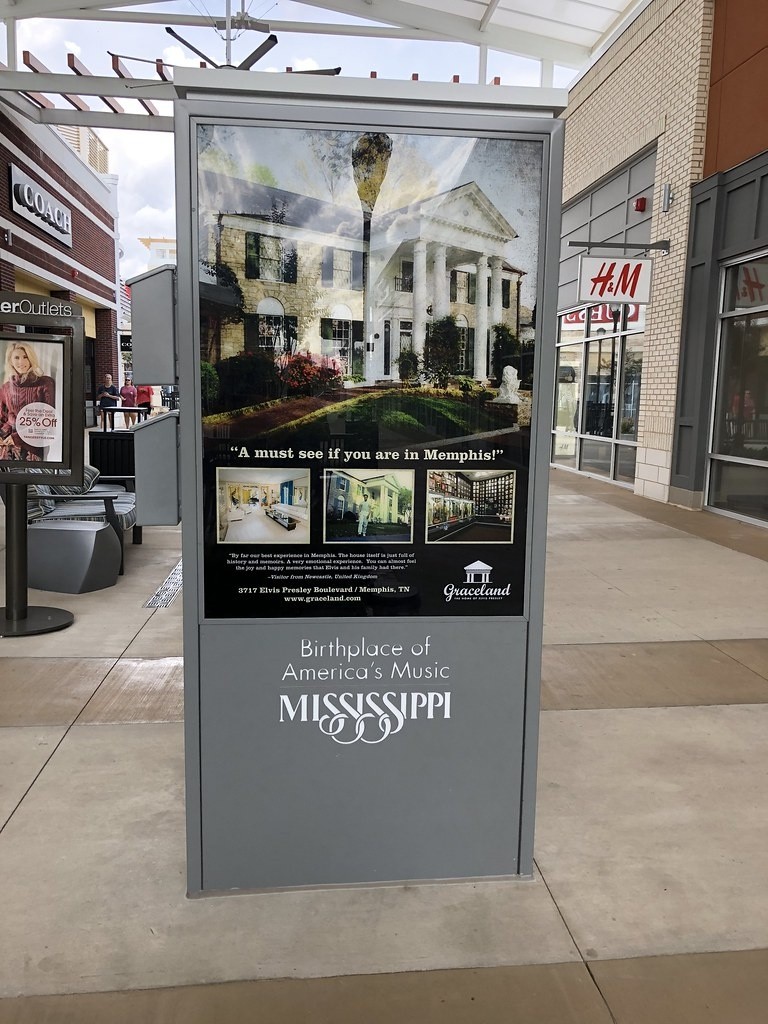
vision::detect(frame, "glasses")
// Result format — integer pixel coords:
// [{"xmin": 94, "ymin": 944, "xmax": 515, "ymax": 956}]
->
[{"xmin": 126, "ymin": 380, "xmax": 130, "ymax": 381}]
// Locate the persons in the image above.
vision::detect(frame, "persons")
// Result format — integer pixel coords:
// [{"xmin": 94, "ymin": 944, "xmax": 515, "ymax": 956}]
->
[
  {"xmin": 358, "ymin": 494, "xmax": 371, "ymax": 536},
  {"xmin": 0, "ymin": 341, "xmax": 56, "ymax": 460},
  {"xmin": 120, "ymin": 377, "xmax": 154, "ymax": 429},
  {"xmin": 98, "ymin": 374, "xmax": 119, "ymax": 428}
]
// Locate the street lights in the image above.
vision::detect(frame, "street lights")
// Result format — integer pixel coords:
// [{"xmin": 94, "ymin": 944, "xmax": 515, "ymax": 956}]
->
[
  {"xmin": 596, "ymin": 328, "xmax": 607, "ymax": 403},
  {"xmin": 609, "ymin": 303, "xmax": 621, "ymax": 405},
  {"xmin": 352, "ymin": 132, "xmax": 393, "ymax": 347}
]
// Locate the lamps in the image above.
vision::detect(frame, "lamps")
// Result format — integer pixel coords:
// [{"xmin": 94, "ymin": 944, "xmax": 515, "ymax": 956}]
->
[
  {"xmin": 662, "ymin": 184, "xmax": 675, "ymax": 211},
  {"xmin": 4, "ymin": 229, "xmax": 13, "ymax": 247}
]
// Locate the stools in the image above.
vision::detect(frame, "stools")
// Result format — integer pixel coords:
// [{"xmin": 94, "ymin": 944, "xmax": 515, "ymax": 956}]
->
[{"xmin": 28, "ymin": 519, "xmax": 122, "ymax": 595}]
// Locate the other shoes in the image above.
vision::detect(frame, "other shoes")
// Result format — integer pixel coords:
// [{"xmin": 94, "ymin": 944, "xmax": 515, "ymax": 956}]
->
[
  {"xmin": 359, "ymin": 533, "xmax": 361, "ymax": 534},
  {"xmin": 363, "ymin": 534, "xmax": 365, "ymax": 536}
]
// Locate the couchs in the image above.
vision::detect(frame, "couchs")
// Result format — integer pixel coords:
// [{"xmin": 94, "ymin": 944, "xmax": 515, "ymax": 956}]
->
[{"xmin": 1, "ymin": 466, "xmax": 142, "ymax": 576}]
